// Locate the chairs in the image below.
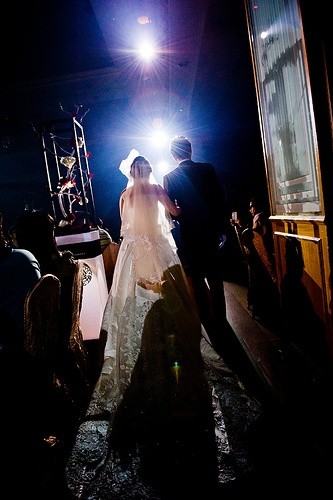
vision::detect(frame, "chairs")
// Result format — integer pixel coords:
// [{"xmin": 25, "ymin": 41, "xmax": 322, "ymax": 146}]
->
[
  {"xmin": 231, "ymin": 219, "xmax": 277, "ymax": 319},
  {"xmin": 69, "ymin": 260, "xmax": 86, "ymax": 379},
  {"xmin": 21, "ymin": 274, "xmax": 62, "ymax": 363}
]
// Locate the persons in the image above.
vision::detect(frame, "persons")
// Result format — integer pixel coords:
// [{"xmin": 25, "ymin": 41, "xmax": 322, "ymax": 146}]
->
[
  {"xmin": 230, "ymin": 198, "xmax": 277, "ymax": 289},
  {"xmin": 0, "ymin": 208, "xmax": 79, "ymax": 403},
  {"xmin": 118, "ymin": 155, "xmax": 182, "ymax": 347},
  {"xmin": 163, "ymin": 136, "xmax": 225, "ymax": 349}
]
